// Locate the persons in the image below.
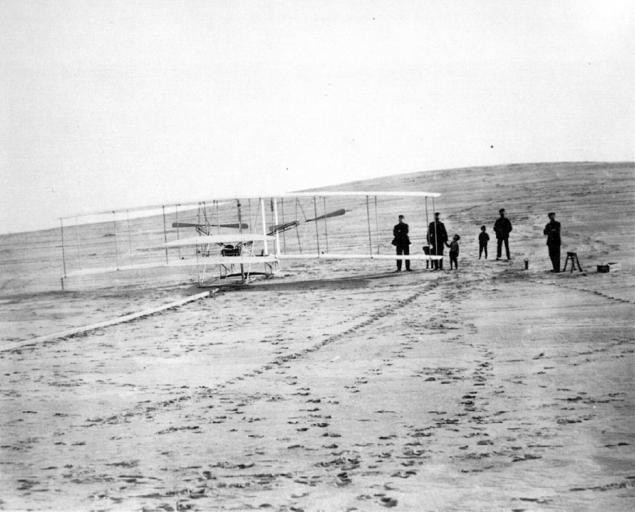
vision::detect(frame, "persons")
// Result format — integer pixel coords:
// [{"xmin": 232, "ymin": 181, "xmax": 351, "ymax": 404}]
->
[
  {"xmin": 478, "ymin": 225, "xmax": 489, "ymax": 260},
  {"xmin": 446, "ymin": 234, "xmax": 461, "ymax": 270},
  {"xmin": 391, "ymin": 215, "xmax": 413, "ymax": 272},
  {"xmin": 493, "ymin": 208, "xmax": 514, "ymax": 260},
  {"xmin": 426, "ymin": 212, "xmax": 448, "ymax": 270},
  {"xmin": 543, "ymin": 211, "xmax": 562, "ymax": 273}
]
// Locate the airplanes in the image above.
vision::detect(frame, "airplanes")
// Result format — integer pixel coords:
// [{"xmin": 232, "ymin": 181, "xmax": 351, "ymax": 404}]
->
[{"xmin": 51, "ymin": 185, "xmax": 444, "ymax": 291}]
